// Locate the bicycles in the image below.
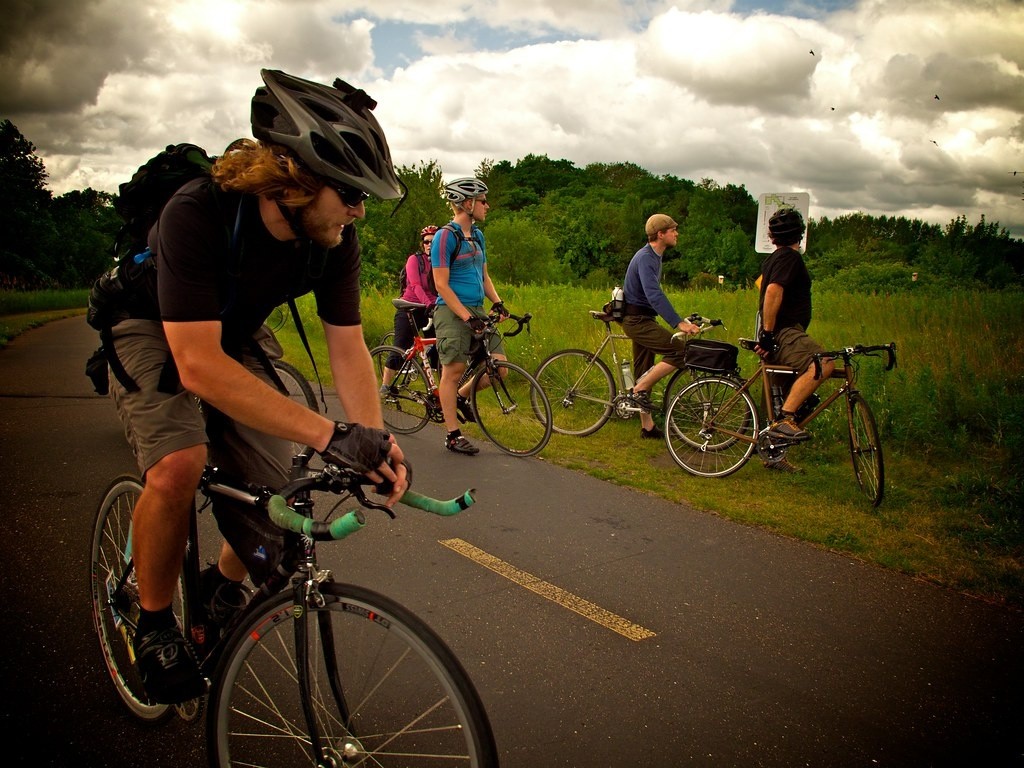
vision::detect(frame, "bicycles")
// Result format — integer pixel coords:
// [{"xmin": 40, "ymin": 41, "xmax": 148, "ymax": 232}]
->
[
  {"xmin": 527, "ymin": 310, "xmax": 754, "ymax": 453},
  {"xmin": 266, "ymin": 354, "xmax": 322, "ymax": 469},
  {"xmin": 663, "ymin": 337, "xmax": 899, "ymax": 510},
  {"xmin": 87, "ymin": 393, "xmax": 500, "ymax": 768},
  {"xmin": 367, "ymin": 299, "xmax": 554, "ymax": 458}
]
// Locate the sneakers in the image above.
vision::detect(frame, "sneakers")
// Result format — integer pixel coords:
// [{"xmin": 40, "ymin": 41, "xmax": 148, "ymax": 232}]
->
[
  {"xmin": 445, "ymin": 434, "xmax": 479, "ymax": 455},
  {"xmin": 768, "ymin": 415, "xmax": 815, "ymax": 440},
  {"xmin": 626, "ymin": 388, "xmax": 661, "ymax": 411},
  {"xmin": 763, "ymin": 451, "xmax": 806, "ymax": 475},
  {"xmin": 640, "ymin": 424, "xmax": 676, "ymax": 440},
  {"xmin": 457, "ymin": 392, "xmax": 477, "ymax": 423}
]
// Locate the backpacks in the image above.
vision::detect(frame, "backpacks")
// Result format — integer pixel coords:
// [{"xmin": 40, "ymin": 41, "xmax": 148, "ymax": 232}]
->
[{"xmin": 109, "ymin": 143, "xmax": 330, "ymax": 340}]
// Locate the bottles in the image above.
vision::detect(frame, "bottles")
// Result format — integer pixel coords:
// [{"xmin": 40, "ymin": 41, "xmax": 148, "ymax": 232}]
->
[
  {"xmin": 621, "ymin": 359, "xmax": 635, "ymax": 390},
  {"xmin": 611, "ymin": 285, "xmax": 623, "ymax": 317},
  {"xmin": 85, "ymin": 265, "xmax": 134, "ymax": 332},
  {"xmin": 770, "ymin": 383, "xmax": 785, "ymax": 421}
]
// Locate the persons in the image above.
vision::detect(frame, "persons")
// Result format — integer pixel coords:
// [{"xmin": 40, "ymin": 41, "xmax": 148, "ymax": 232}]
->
[
  {"xmin": 430, "ymin": 177, "xmax": 509, "ymax": 455},
  {"xmin": 754, "ymin": 209, "xmax": 834, "ymax": 474},
  {"xmin": 99, "ymin": 68, "xmax": 414, "ymax": 704},
  {"xmin": 379, "ymin": 225, "xmax": 442, "ymax": 398},
  {"xmin": 619, "ymin": 214, "xmax": 698, "ymax": 439}
]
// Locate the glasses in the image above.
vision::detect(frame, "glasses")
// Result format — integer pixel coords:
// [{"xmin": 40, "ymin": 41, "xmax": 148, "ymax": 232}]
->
[
  {"xmin": 420, "ymin": 239, "xmax": 432, "ymax": 245},
  {"xmin": 472, "ymin": 198, "xmax": 491, "ymax": 207},
  {"xmin": 274, "ymin": 152, "xmax": 370, "ymax": 209}
]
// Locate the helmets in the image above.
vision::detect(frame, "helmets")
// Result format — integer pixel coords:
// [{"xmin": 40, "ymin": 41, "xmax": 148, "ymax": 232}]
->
[
  {"xmin": 444, "ymin": 178, "xmax": 489, "ymax": 203},
  {"xmin": 251, "ymin": 68, "xmax": 402, "ymax": 203},
  {"xmin": 420, "ymin": 225, "xmax": 440, "ymax": 235}
]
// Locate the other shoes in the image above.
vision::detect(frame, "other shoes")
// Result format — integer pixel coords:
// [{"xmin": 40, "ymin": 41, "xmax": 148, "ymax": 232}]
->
[
  {"xmin": 196, "ymin": 562, "xmax": 256, "ymax": 657},
  {"xmin": 414, "ymin": 364, "xmax": 432, "ymax": 375},
  {"xmin": 132, "ymin": 611, "xmax": 209, "ymax": 706},
  {"xmin": 379, "ymin": 385, "xmax": 397, "ymax": 402}
]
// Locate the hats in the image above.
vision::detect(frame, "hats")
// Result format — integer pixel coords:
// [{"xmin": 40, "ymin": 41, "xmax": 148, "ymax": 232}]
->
[{"xmin": 644, "ymin": 214, "xmax": 679, "ymax": 235}]
